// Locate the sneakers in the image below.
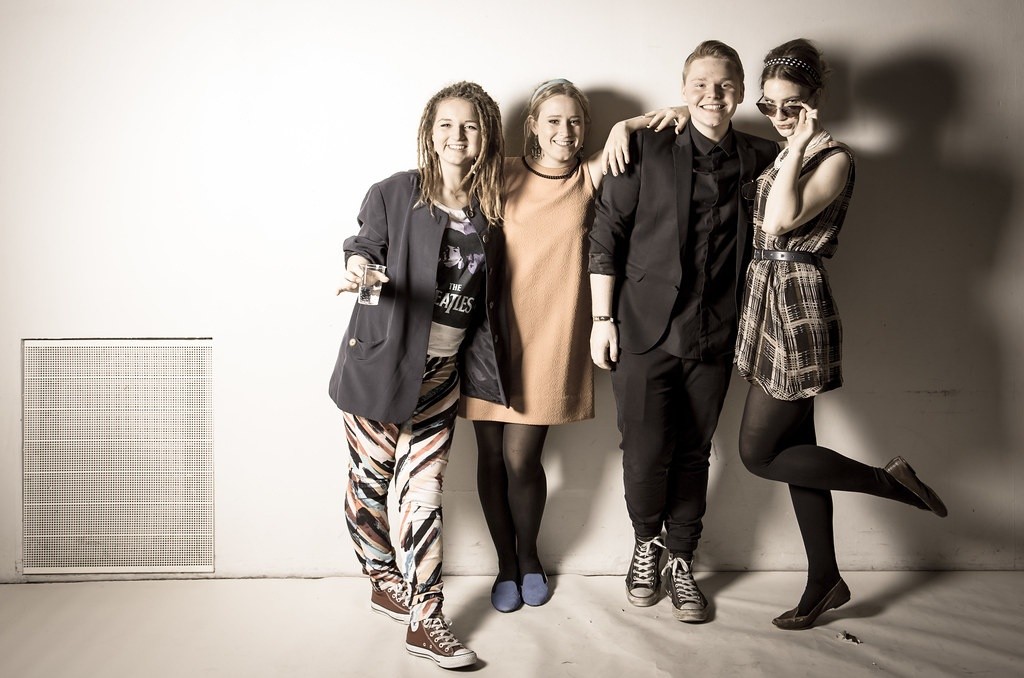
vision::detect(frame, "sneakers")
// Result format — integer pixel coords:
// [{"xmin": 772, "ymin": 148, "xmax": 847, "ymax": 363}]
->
[
  {"xmin": 625, "ymin": 535, "xmax": 667, "ymax": 608},
  {"xmin": 370, "ymin": 585, "xmax": 415, "ymax": 624},
  {"xmin": 661, "ymin": 553, "xmax": 709, "ymax": 623},
  {"xmin": 405, "ymin": 609, "xmax": 477, "ymax": 669}
]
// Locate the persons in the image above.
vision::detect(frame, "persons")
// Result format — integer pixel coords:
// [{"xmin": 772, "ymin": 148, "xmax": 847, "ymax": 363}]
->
[
  {"xmin": 329, "ymin": 83, "xmax": 510, "ymax": 672},
  {"xmin": 457, "ymin": 79, "xmax": 672, "ymax": 614},
  {"xmin": 587, "ymin": 41, "xmax": 780, "ymax": 624},
  {"xmin": 644, "ymin": 37, "xmax": 947, "ymax": 630}
]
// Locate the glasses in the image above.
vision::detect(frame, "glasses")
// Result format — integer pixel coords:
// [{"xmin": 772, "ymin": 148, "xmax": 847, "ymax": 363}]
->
[{"xmin": 755, "ymin": 89, "xmax": 817, "ymax": 119}]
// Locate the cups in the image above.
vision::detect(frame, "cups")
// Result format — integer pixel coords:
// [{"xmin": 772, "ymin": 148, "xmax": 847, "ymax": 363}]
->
[{"xmin": 357, "ymin": 263, "xmax": 387, "ymax": 306}]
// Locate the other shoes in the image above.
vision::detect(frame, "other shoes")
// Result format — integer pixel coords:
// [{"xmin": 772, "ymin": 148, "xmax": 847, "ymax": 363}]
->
[
  {"xmin": 883, "ymin": 456, "xmax": 948, "ymax": 518},
  {"xmin": 519, "ymin": 560, "xmax": 551, "ymax": 607},
  {"xmin": 772, "ymin": 576, "xmax": 851, "ymax": 630},
  {"xmin": 491, "ymin": 572, "xmax": 522, "ymax": 613}
]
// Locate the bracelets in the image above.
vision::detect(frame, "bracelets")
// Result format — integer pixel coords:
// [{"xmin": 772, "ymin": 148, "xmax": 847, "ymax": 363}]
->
[{"xmin": 592, "ymin": 316, "xmax": 613, "ymax": 321}]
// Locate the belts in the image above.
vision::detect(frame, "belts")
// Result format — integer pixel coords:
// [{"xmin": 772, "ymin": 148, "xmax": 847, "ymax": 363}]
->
[{"xmin": 753, "ymin": 248, "xmax": 818, "ymax": 265}]
